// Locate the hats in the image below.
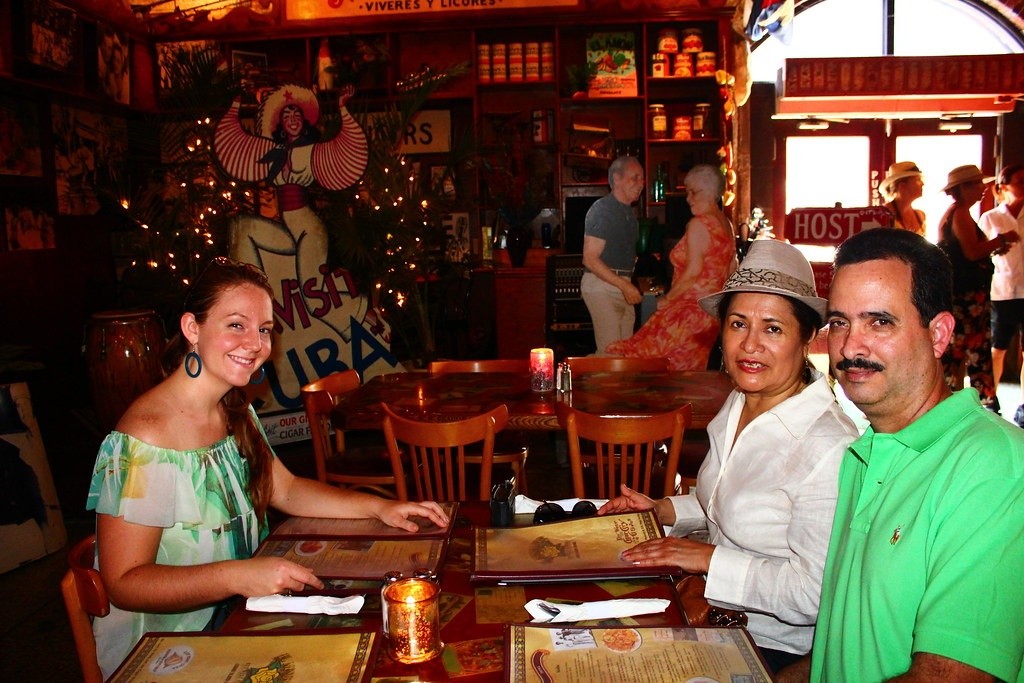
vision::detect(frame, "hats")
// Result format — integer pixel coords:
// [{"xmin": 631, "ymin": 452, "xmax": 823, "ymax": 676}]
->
[
  {"xmin": 938, "ymin": 164, "xmax": 996, "ymax": 193},
  {"xmin": 697, "ymin": 238, "xmax": 828, "ymax": 330},
  {"xmin": 878, "ymin": 161, "xmax": 924, "ymax": 195}
]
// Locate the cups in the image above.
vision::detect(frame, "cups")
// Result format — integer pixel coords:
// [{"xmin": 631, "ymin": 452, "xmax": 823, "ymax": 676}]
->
[{"xmin": 652, "ymin": 60, "xmax": 664, "ymax": 77}]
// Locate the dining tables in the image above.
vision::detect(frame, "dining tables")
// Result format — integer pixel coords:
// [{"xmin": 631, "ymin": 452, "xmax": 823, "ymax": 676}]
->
[{"xmin": 199, "ymin": 498, "xmax": 781, "ymax": 683}]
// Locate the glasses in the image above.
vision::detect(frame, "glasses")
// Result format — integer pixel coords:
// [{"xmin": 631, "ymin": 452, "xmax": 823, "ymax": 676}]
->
[
  {"xmin": 183, "ymin": 257, "xmax": 268, "ymax": 306},
  {"xmin": 532, "ymin": 499, "xmax": 597, "ymax": 523}
]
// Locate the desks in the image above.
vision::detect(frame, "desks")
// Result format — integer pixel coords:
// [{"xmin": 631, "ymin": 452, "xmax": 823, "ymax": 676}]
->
[{"xmin": 335, "ymin": 368, "xmax": 736, "ymax": 455}]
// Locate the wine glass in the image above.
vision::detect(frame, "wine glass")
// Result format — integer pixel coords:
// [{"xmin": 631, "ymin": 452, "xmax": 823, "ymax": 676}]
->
[{"xmin": 483, "ymin": 111, "xmax": 522, "ymax": 144}]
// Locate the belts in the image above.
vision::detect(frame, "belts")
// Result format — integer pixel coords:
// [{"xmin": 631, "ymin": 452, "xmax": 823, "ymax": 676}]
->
[{"xmin": 609, "ymin": 270, "xmax": 634, "ymax": 277}]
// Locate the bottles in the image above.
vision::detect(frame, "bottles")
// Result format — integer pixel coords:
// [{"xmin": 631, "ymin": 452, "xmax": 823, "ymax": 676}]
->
[
  {"xmin": 317, "ymin": 40, "xmax": 333, "ymax": 90},
  {"xmin": 654, "ymin": 165, "xmax": 664, "ymax": 203},
  {"xmin": 557, "ymin": 362, "xmax": 571, "ymax": 392},
  {"xmin": 616, "ymin": 144, "xmax": 641, "ymax": 160},
  {"xmin": 542, "ymin": 223, "xmax": 560, "ymax": 246},
  {"xmin": 478, "ymin": 31, "xmax": 554, "ymax": 80},
  {"xmin": 649, "ymin": 102, "xmax": 711, "ymax": 139},
  {"xmin": 382, "ymin": 571, "xmax": 404, "ymax": 633},
  {"xmin": 413, "ymin": 568, "xmax": 431, "ymax": 582},
  {"xmin": 498, "ymin": 225, "xmax": 508, "ymax": 249},
  {"xmin": 657, "ymin": 28, "xmax": 715, "ymax": 76},
  {"xmin": 555, "ymin": 264, "xmax": 585, "ymax": 298}
]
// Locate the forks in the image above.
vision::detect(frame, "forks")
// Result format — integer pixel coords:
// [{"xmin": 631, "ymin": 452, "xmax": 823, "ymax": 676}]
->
[{"xmin": 542, "ymin": 602, "xmax": 560, "ymax": 612}]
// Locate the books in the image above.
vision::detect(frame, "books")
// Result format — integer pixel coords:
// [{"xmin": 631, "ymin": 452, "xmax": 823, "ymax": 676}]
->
[
  {"xmin": 105, "ymin": 628, "xmax": 382, "ymax": 683},
  {"xmin": 248, "ymin": 502, "xmax": 458, "ymax": 579},
  {"xmin": 470, "ymin": 509, "xmax": 667, "ymax": 579},
  {"xmin": 505, "ymin": 621, "xmax": 777, "ymax": 683}
]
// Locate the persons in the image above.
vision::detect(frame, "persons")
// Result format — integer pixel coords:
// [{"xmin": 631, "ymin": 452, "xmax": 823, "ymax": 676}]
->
[
  {"xmin": 0, "ymin": 10, "xmax": 129, "ymax": 253},
  {"xmin": 938, "ymin": 159, "xmax": 1024, "ymax": 420},
  {"xmin": 878, "ymin": 161, "xmax": 925, "ymax": 239},
  {"xmin": 603, "ymin": 161, "xmax": 738, "ymax": 371},
  {"xmin": 85, "ymin": 257, "xmax": 450, "ymax": 683},
  {"xmin": 581, "ymin": 155, "xmax": 645, "ymax": 351},
  {"xmin": 597, "ymin": 227, "xmax": 1024, "ymax": 683}
]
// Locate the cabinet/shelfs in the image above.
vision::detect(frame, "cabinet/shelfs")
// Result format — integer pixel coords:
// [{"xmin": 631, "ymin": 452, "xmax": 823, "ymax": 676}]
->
[{"xmin": 156, "ymin": 18, "xmax": 741, "ymax": 268}]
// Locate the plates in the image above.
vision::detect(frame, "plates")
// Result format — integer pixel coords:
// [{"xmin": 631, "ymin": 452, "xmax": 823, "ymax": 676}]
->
[
  {"xmin": 687, "ymin": 677, "xmax": 720, "ymax": 683},
  {"xmin": 295, "ymin": 540, "xmax": 327, "ymax": 557},
  {"xmin": 603, "ymin": 629, "xmax": 641, "ymax": 653}
]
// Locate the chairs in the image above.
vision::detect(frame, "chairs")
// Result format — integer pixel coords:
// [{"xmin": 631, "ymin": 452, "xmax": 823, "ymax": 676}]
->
[
  {"xmin": 298, "ymin": 368, "xmax": 422, "ymax": 501},
  {"xmin": 376, "ymin": 402, "xmax": 525, "ymax": 504},
  {"xmin": 59, "ymin": 534, "xmax": 112, "ymax": 683},
  {"xmin": 563, "ymin": 355, "xmax": 678, "ymax": 469},
  {"xmin": 429, "ymin": 357, "xmax": 538, "ymax": 497},
  {"xmin": 553, "ymin": 399, "xmax": 695, "ymax": 498}
]
